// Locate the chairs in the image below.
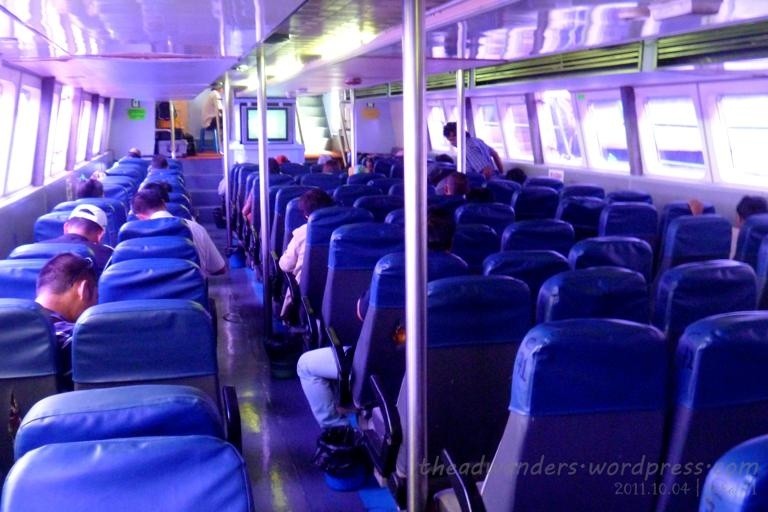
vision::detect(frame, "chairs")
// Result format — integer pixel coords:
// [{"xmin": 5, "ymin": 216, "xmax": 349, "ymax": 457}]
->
[
  {"xmin": 0, "ymin": 297, "xmax": 63, "ymax": 486},
  {"xmin": 73, "ymin": 299, "xmax": 222, "ymax": 406},
  {"xmin": 12, "ymin": 384, "xmax": 243, "ymax": 458},
  {"xmin": 0, "ymin": 435, "xmax": 255, "ymax": 512},
  {"xmin": 355, "ymin": 275, "xmax": 534, "ymax": 511},
  {"xmin": 432, "ymin": 317, "xmax": 666, "ymax": 512},
  {"xmin": 325, "ymin": 252, "xmax": 467, "ymax": 414},
  {"xmin": 654, "ymin": 311, "xmax": 767, "ymax": 512},
  {"xmin": 699, "ymin": 436, "xmax": 768, "ymax": 512}
]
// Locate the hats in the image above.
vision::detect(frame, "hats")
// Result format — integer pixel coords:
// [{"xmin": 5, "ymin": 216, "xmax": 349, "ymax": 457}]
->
[{"xmin": 67, "ymin": 204, "xmax": 109, "ymax": 233}]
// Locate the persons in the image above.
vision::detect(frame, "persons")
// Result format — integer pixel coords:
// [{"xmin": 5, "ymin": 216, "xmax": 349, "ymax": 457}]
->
[
  {"xmin": 33, "ymin": 252, "xmax": 98, "ymax": 365},
  {"xmin": 432, "ymin": 122, "xmax": 527, "ymax": 196},
  {"xmin": 158, "ymin": 112, "xmax": 197, "ymax": 156},
  {"xmin": 201, "ymin": 80, "xmax": 224, "ymax": 129},
  {"xmin": 131, "ymin": 190, "xmax": 227, "ymax": 280},
  {"xmin": 278, "ymin": 189, "xmax": 335, "ymax": 285},
  {"xmin": 76, "ymin": 147, "xmax": 170, "ymax": 202},
  {"xmin": 686, "ymin": 194, "xmax": 768, "ymax": 260},
  {"xmin": 296, "ymin": 207, "xmax": 456, "ymax": 430},
  {"xmin": 34, "ymin": 204, "xmax": 114, "ymax": 255},
  {"xmin": 242, "ymin": 155, "xmax": 373, "ymax": 224}
]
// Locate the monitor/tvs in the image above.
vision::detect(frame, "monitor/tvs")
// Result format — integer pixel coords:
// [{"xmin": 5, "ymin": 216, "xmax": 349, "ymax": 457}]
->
[{"xmin": 240, "ymin": 104, "xmax": 292, "ymax": 144}]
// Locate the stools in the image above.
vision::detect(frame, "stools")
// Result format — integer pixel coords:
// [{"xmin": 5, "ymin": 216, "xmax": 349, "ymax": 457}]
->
[{"xmin": 199, "ymin": 128, "xmax": 219, "ymax": 153}]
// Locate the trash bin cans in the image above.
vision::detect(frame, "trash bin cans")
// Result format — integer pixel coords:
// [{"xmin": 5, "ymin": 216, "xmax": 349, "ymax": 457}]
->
[
  {"xmin": 312, "ymin": 426, "xmax": 370, "ymax": 490},
  {"xmin": 213, "ymin": 208, "xmax": 226, "ymax": 228},
  {"xmin": 224, "ymin": 246, "xmax": 246, "ymax": 268},
  {"xmin": 272, "ymin": 298, "xmax": 290, "ymax": 329}
]
